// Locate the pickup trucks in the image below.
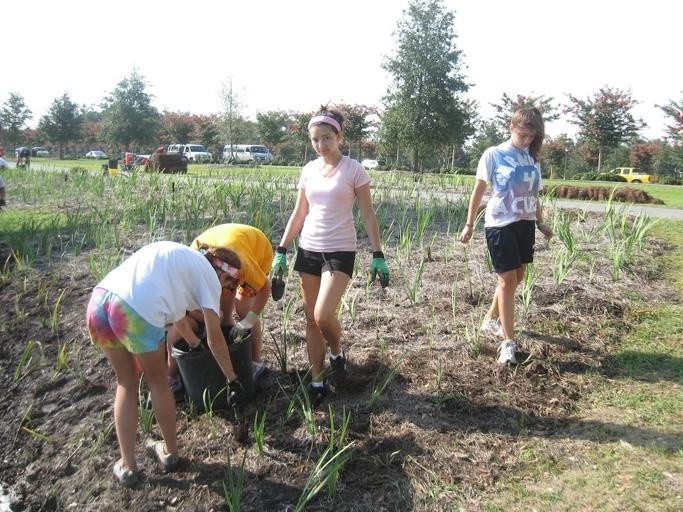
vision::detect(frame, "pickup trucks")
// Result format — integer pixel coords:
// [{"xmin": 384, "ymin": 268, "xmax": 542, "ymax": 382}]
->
[{"xmin": 598, "ymin": 165, "xmax": 659, "ymax": 185}]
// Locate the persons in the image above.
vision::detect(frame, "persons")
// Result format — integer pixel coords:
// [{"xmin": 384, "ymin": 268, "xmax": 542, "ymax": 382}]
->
[
  {"xmin": 457, "ymin": 103, "xmax": 554, "ymax": 366},
  {"xmin": 0, "ymin": 147, "xmax": 10, "ymax": 208},
  {"xmin": 164, "ymin": 222, "xmax": 274, "ymax": 392},
  {"xmin": 86, "ymin": 239, "xmax": 250, "ymax": 488},
  {"xmin": 269, "ymin": 109, "xmax": 390, "ymax": 407},
  {"xmin": 15, "ymin": 146, "xmax": 31, "ymax": 169}
]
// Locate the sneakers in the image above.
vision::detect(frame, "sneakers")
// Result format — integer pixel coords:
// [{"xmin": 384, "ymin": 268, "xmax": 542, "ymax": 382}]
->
[
  {"xmin": 480, "ymin": 317, "xmax": 504, "ymax": 339},
  {"xmin": 329, "ymin": 352, "xmax": 348, "ymax": 389},
  {"xmin": 495, "ymin": 340, "xmax": 517, "ymax": 366},
  {"xmin": 146, "ymin": 375, "xmax": 182, "ymax": 404},
  {"xmin": 251, "ymin": 360, "xmax": 265, "ymax": 383},
  {"xmin": 155, "ymin": 443, "xmax": 180, "ymax": 467},
  {"xmin": 302, "ymin": 379, "xmax": 327, "ymax": 406},
  {"xmin": 114, "ymin": 458, "xmax": 139, "ymax": 486}
]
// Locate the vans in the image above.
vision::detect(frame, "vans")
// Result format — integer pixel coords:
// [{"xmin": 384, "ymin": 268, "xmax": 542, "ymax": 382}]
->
[
  {"xmin": 167, "ymin": 143, "xmax": 212, "ymax": 163},
  {"xmin": 222, "ymin": 144, "xmax": 273, "ymax": 162}
]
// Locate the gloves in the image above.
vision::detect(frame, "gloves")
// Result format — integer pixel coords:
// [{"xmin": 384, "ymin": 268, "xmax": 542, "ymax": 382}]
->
[
  {"xmin": 225, "ymin": 378, "xmax": 244, "ymax": 407},
  {"xmin": 229, "ymin": 309, "xmax": 259, "ymax": 343},
  {"xmin": 272, "ymin": 247, "xmax": 289, "ymax": 276},
  {"xmin": 369, "ymin": 251, "xmax": 390, "ymax": 288}
]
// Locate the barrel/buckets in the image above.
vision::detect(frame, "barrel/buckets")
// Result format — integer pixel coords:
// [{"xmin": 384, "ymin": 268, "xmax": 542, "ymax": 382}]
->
[{"xmin": 170, "ymin": 326, "xmax": 256, "ymax": 414}]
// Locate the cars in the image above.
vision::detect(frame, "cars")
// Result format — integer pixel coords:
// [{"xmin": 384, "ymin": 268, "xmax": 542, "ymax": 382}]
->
[
  {"xmin": 86, "ymin": 151, "xmax": 106, "ymax": 157},
  {"xmin": 14, "ymin": 146, "xmax": 51, "ymax": 157},
  {"xmin": 362, "ymin": 159, "xmax": 385, "ymax": 169}
]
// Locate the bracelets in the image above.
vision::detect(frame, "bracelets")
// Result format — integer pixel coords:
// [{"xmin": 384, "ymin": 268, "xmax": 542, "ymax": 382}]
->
[
  {"xmin": 464, "ymin": 222, "xmax": 473, "ymax": 227},
  {"xmin": 537, "ymin": 220, "xmax": 545, "ymax": 230}
]
